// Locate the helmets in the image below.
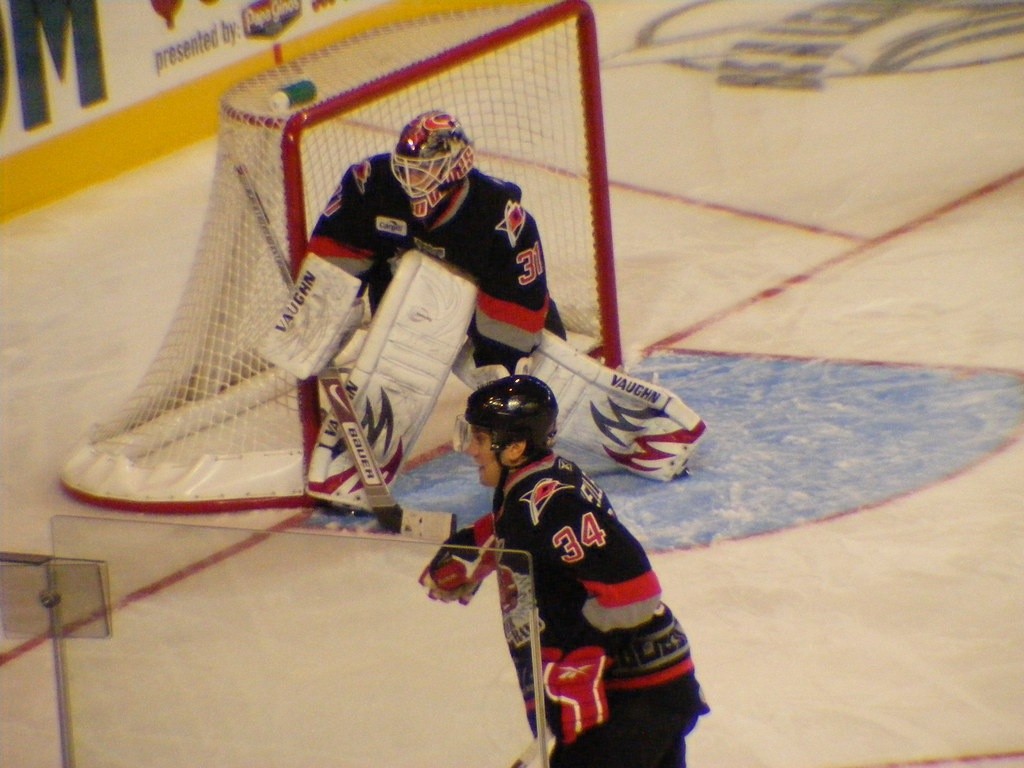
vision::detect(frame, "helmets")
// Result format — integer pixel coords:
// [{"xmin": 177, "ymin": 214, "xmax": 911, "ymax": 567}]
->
[
  {"xmin": 464, "ymin": 375, "xmax": 559, "ymax": 462},
  {"xmin": 396, "ymin": 112, "xmax": 473, "ymax": 220}
]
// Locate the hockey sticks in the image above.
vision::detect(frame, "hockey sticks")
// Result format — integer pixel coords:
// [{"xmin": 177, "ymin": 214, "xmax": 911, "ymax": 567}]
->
[
  {"xmin": 218, "ymin": 127, "xmax": 459, "ymax": 543},
  {"xmin": 512, "ymin": 725, "xmax": 555, "ymax": 768}
]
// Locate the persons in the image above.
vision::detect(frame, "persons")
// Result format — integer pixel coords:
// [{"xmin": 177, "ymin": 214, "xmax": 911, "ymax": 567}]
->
[
  {"xmin": 419, "ymin": 375, "xmax": 710, "ymax": 768},
  {"xmin": 259, "ymin": 111, "xmax": 706, "ymax": 518}
]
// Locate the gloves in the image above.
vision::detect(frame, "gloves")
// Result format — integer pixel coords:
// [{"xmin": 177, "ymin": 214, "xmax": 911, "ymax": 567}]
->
[
  {"xmin": 540, "ymin": 647, "xmax": 614, "ymax": 749},
  {"xmin": 418, "ymin": 514, "xmax": 498, "ymax": 605}
]
